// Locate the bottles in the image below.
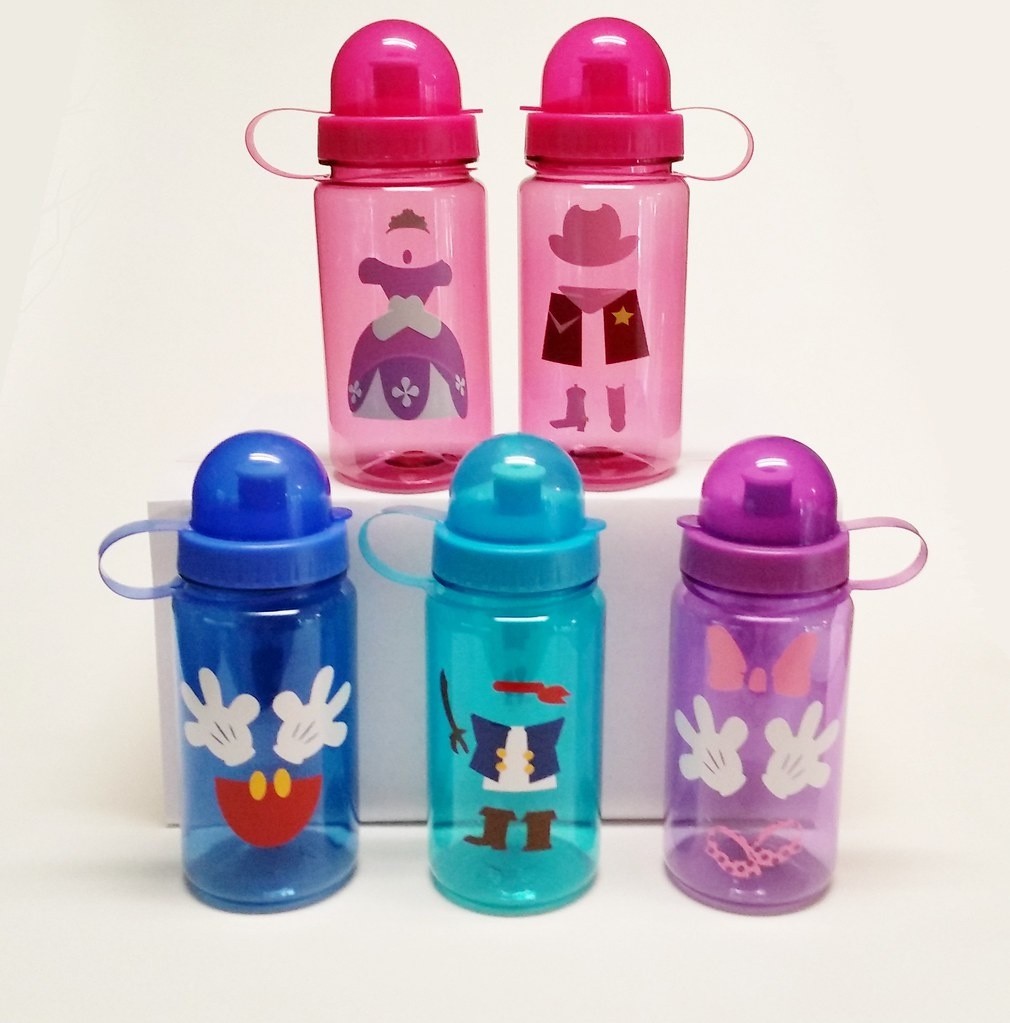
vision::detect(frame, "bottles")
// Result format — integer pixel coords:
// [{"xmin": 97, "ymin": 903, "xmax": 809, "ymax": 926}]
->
[
  {"xmin": 245, "ymin": 19, "xmax": 494, "ymax": 495},
  {"xmin": 663, "ymin": 435, "xmax": 928, "ymax": 916},
  {"xmin": 514, "ymin": 16, "xmax": 755, "ymax": 492},
  {"xmin": 96, "ymin": 430, "xmax": 357, "ymax": 914},
  {"xmin": 356, "ymin": 433, "xmax": 607, "ymax": 919}
]
